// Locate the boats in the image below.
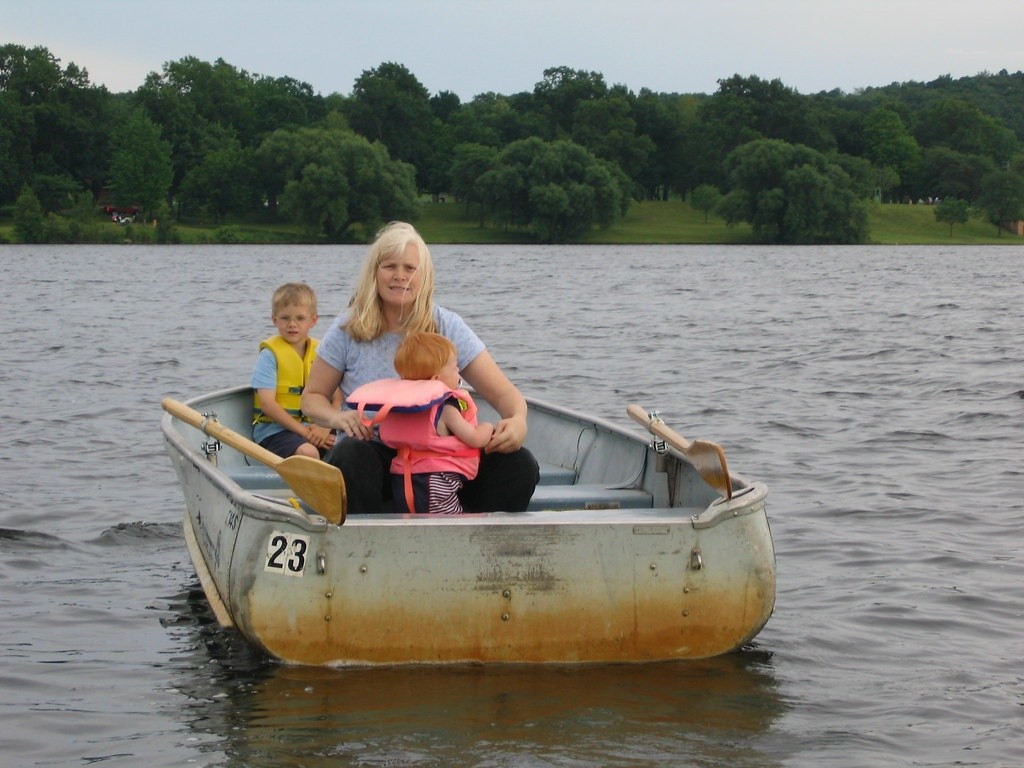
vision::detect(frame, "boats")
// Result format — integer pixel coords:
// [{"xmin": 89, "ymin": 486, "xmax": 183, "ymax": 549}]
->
[{"xmin": 158, "ymin": 381, "xmax": 776, "ymax": 676}]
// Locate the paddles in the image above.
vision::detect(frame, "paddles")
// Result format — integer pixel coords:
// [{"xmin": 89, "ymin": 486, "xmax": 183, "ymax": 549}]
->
[
  {"xmin": 161, "ymin": 397, "xmax": 348, "ymax": 527},
  {"xmin": 626, "ymin": 403, "xmax": 733, "ymax": 502}
]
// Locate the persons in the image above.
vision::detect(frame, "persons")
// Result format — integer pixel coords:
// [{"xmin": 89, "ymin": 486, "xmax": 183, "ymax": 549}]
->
[
  {"xmin": 300, "ymin": 220, "xmax": 540, "ymax": 513},
  {"xmin": 346, "ymin": 332, "xmax": 495, "ymax": 514},
  {"xmin": 251, "ymin": 281, "xmax": 343, "ymax": 460}
]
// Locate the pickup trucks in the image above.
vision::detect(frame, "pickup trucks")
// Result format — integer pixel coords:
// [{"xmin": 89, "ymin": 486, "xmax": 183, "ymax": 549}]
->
[{"xmin": 100, "ymin": 205, "xmax": 141, "ymax": 216}]
